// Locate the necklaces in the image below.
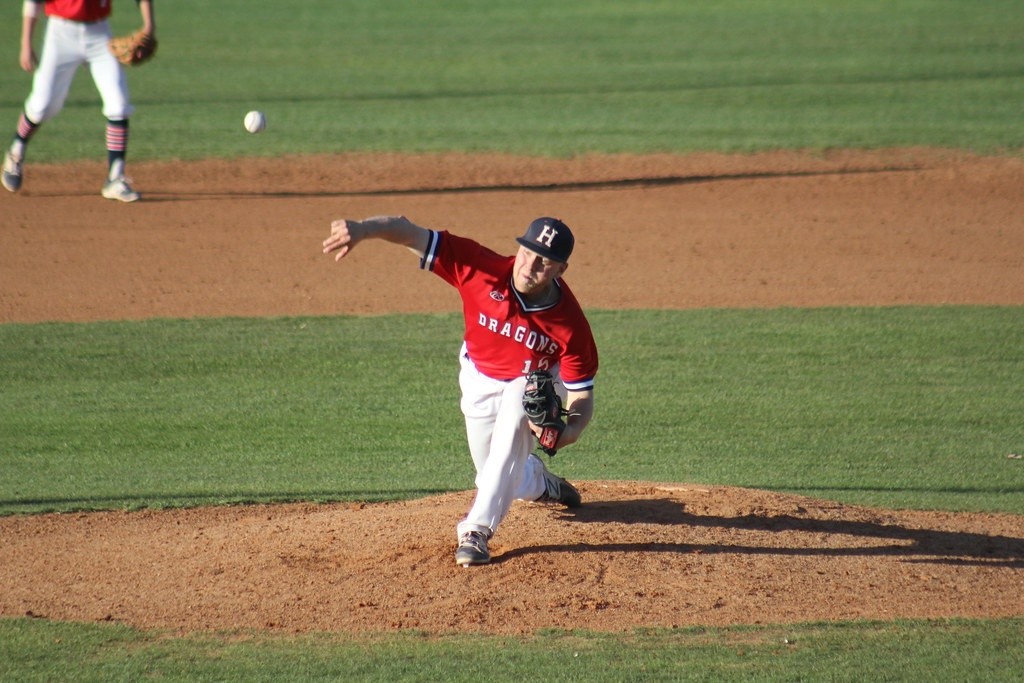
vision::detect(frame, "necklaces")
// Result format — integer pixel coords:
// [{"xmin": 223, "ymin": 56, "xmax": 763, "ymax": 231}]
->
[{"xmin": 524, "ymin": 285, "xmax": 552, "ymax": 307}]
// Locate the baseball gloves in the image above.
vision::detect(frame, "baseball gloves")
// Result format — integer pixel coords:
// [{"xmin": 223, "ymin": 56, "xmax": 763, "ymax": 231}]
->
[
  {"xmin": 521, "ymin": 368, "xmax": 569, "ymax": 458},
  {"xmin": 105, "ymin": 31, "xmax": 159, "ymax": 69}
]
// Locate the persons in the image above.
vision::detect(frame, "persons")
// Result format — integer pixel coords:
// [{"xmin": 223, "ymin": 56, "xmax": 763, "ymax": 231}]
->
[
  {"xmin": 0, "ymin": 0, "xmax": 158, "ymax": 203},
  {"xmin": 323, "ymin": 214, "xmax": 599, "ymax": 565}
]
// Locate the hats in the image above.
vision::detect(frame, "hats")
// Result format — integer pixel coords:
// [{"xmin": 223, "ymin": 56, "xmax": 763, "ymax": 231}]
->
[{"xmin": 517, "ymin": 217, "xmax": 575, "ymax": 263}]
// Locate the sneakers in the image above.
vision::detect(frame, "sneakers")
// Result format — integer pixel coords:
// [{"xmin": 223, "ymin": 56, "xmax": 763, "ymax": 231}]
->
[
  {"xmin": 532, "ymin": 453, "xmax": 582, "ymax": 507},
  {"xmin": 102, "ymin": 175, "xmax": 141, "ymax": 202},
  {"xmin": 455, "ymin": 530, "xmax": 490, "ymax": 564},
  {"xmin": 1, "ymin": 150, "xmax": 25, "ymax": 192}
]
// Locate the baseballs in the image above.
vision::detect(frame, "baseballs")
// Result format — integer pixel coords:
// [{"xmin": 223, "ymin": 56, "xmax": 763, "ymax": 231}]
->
[{"xmin": 241, "ymin": 109, "xmax": 269, "ymax": 135}]
